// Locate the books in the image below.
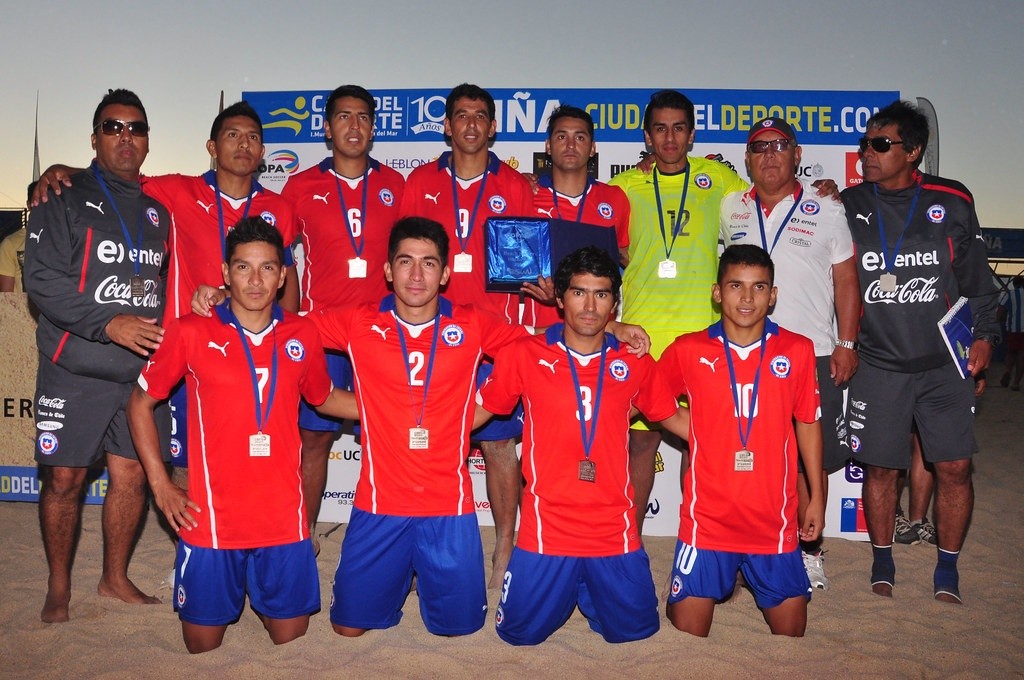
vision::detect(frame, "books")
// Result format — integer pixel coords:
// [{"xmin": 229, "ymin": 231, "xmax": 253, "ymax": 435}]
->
[{"xmin": 937, "ymin": 296, "xmax": 974, "ymax": 379}]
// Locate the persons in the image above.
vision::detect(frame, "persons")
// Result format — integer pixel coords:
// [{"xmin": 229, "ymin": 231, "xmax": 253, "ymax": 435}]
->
[
  {"xmin": 636, "ymin": 116, "xmax": 862, "ymax": 591},
  {"xmin": 630, "ymin": 244, "xmax": 826, "ymax": 638},
  {"xmin": 24, "ymin": 89, "xmax": 169, "ymax": 623},
  {"xmin": 471, "ymin": 244, "xmax": 689, "ymax": 646},
  {"xmin": 520, "ymin": 106, "xmax": 630, "ymax": 328},
  {"xmin": 521, "ymin": 89, "xmax": 842, "ymax": 536},
  {"xmin": 0, "ymin": 181, "xmax": 38, "ymax": 294},
  {"xmin": 31, "ymin": 100, "xmax": 300, "ymax": 589},
  {"xmin": 399, "ymin": 84, "xmax": 556, "ymax": 588},
  {"xmin": 838, "ymin": 99, "xmax": 1002, "ymax": 605},
  {"xmin": 125, "ymin": 216, "xmax": 360, "ymax": 654},
  {"xmin": 190, "ymin": 215, "xmax": 651, "ymax": 638},
  {"xmin": 281, "ymin": 85, "xmax": 406, "ymax": 557}
]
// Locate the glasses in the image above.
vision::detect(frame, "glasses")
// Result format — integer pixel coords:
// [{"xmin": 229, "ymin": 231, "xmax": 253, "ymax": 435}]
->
[
  {"xmin": 749, "ymin": 138, "xmax": 789, "ymax": 154},
  {"xmin": 93, "ymin": 119, "xmax": 149, "ymax": 136},
  {"xmin": 859, "ymin": 137, "xmax": 903, "ymax": 153}
]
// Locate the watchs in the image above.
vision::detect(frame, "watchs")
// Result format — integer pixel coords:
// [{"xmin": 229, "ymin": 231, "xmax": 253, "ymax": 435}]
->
[{"xmin": 835, "ymin": 339, "xmax": 860, "ymax": 351}]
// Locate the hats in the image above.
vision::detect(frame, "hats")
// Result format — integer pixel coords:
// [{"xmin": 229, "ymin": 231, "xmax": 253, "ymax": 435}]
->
[{"xmin": 746, "ymin": 117, "xmax": 797, "ymax": 152}]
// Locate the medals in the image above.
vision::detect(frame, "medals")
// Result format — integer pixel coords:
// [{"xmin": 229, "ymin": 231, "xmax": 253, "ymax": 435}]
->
[
  {"xmin": 349, "ymin": 259, "xmax": 367, "ymax": 278},
  {"xmin": 880, "ymin": 275, "xmax": 896, "ymax": 292},
  {"xmin": 409, "ymin": 428, "xmax": 429, "ymax": 450},
  {"xmin": 130, "ymin": 278, "xmax": 145, "ymax": 296},
  {"xmin": 578, "ymin": 460, "xmax": 596, "ymax": 482},
  {"xmin": 454, "ymin": 254, "xmax": 472, "ymax": 272},
  {"xmin": 734, "ymin": 451, "xmax": 753, "ymax": 471},
  {"xmin": 249, "ymin": 434, "xmax": 270, "ymax": 456},
  {"xmin": 658, "ymin": 260, "xmax": 676, "ymax": 279}
]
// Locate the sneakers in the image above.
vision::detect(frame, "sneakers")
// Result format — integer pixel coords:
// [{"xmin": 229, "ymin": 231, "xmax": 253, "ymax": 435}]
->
[
  {"xmin": 912, "ymin": 519, "xmax": 937, "ymax": 547},
  {"xmin": 893, "ymin": 510, "xmax": 922, "ymax": 545},
  {"xmin": 801, "ymin": 549, "xmax": 829, "ymax": 590}
]
